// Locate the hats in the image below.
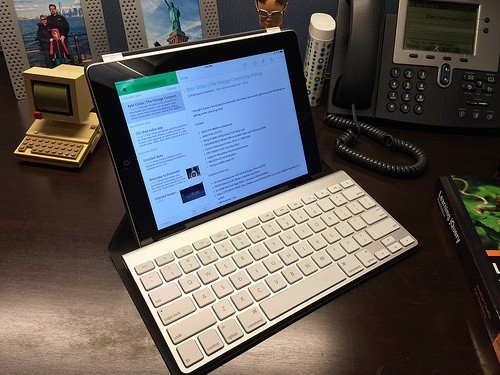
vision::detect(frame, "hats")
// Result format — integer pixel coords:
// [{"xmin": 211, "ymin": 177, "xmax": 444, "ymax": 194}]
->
[{"xmin": 51, "ymin": 28, "xmax": 59, "ymax": 37}]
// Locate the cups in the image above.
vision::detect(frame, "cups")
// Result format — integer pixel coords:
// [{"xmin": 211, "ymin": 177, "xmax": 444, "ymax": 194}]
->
[{"xmin": 305, "ymin": 13, "xmax": 336, "ymax": 106}]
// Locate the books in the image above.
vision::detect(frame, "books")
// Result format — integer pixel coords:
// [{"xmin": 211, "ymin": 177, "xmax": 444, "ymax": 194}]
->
[{"xmin": 433, "ymin": 173, "xmax": 500, "ymax": 364}]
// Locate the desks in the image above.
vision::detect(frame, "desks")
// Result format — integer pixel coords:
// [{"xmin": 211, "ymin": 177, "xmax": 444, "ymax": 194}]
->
[{"xmin": 0, "ymin": 44, "xmax": 499, "ymax": 375}]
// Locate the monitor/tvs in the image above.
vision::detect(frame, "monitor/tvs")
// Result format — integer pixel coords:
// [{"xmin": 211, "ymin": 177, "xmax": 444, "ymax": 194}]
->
[{"xmin": 22, "ymin": 63, "xmax": 95, "ymax": 123}]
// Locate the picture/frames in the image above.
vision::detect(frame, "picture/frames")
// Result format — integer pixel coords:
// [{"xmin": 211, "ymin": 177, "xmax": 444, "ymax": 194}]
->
[
  {"xmin": 0, "ymin": 1, "xmax": 111, "ymax": 100},
  {"xmin": 119, "ymin": 1, "xmax": 221, "ymax": 51}
]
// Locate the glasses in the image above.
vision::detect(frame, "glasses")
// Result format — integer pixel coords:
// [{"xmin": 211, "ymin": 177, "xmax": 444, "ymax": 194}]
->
[{"xmin": 41, "ymin": 18, "xmax": 46, "ymax": 20}]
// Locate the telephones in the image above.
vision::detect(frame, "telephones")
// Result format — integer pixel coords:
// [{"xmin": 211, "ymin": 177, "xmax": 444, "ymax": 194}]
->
[{"xmin": 326, "ymin": 1, "xmax": 500, "ymax": 133}]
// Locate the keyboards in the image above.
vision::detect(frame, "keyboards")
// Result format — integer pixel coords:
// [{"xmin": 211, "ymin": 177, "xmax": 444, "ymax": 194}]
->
[
  {"xmin": 14, "ymin": 135, "xmax": 89, "ymax": 165},
  {"xmin": 121, "ymin": 169, "xmax": 419, "ymax": 375}
]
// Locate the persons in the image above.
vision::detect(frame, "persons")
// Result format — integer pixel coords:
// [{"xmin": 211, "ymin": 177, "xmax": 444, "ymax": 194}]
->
[
  {"xmin": 37, "ymin": 15, "xmax": 61, "ymax": 68},
  {"xmin": 50, "ymin": 28, "xmax": 69, "ymax": 65},
  {"xmin": 164, "ymin": 0, "xmax": 181, "ymax": 31},
  {"xmin": 35, "ymin": 4, "xmax": 75, "ymax": 67}
]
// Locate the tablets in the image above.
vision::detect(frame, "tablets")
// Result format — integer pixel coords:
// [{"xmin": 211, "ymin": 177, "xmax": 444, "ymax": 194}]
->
[{"xmin": 83, "ymin": 26, "xmax": 324, "ymax": 249}]
